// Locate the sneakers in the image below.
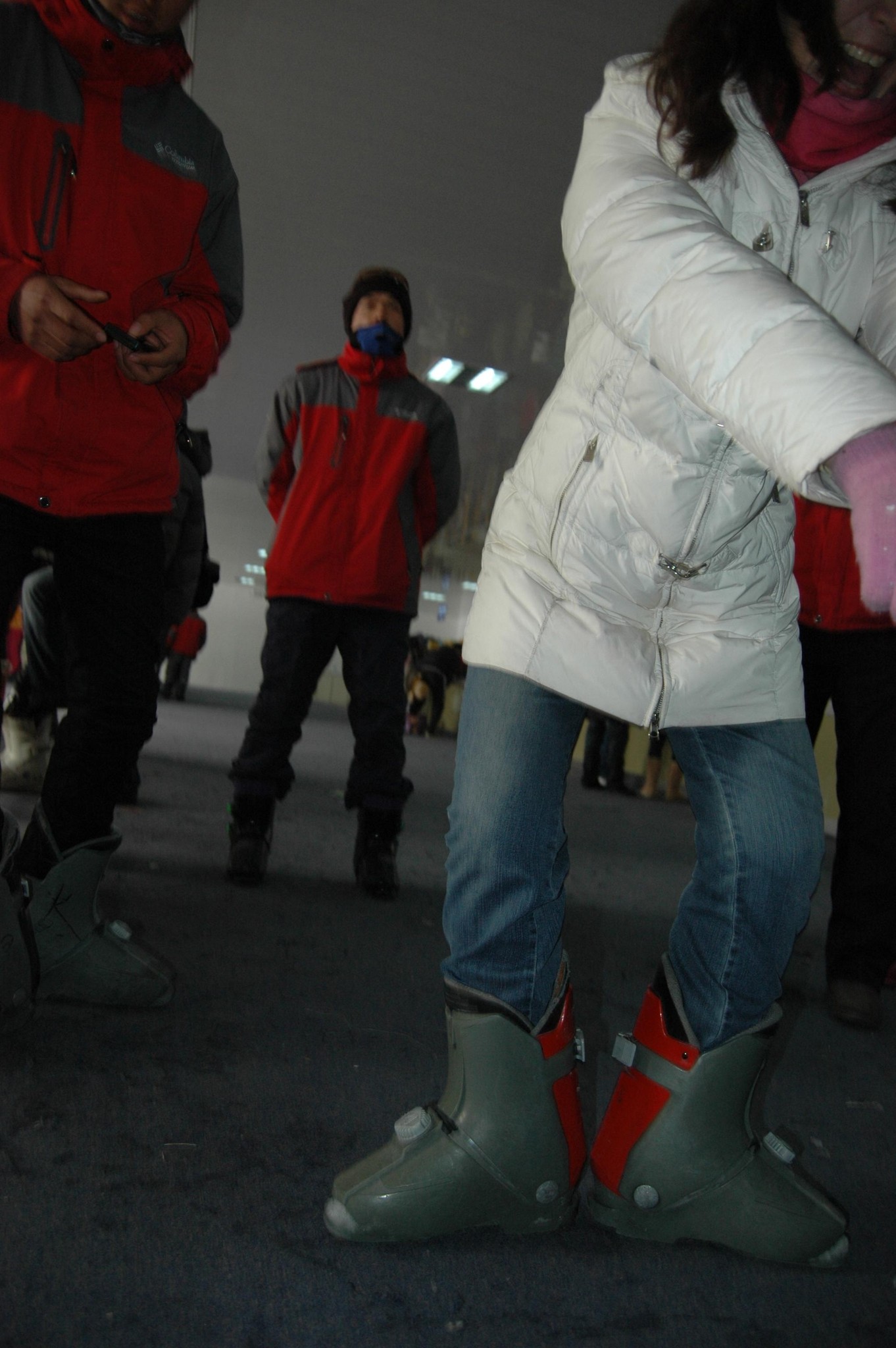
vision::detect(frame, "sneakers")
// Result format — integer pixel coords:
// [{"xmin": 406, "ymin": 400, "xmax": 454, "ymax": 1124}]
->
[
  {"xmin": 323, "ymin": 947, "xmax": 586, "ymax": 1244},
  {"xmin": 582, "ymin": 952, "xmax": 851, "ymax": 1269},
  {"xmin": 0, "ymin": 809, "xmax": 177, "ymax": 1006}
]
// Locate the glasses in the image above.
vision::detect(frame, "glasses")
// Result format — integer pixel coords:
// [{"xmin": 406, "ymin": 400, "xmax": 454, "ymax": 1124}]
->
[{"xmin": 356, "ymin": 268, "xmax": 408, "ymax": 287}]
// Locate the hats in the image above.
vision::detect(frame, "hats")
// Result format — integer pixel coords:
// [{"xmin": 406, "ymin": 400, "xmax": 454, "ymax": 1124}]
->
[{"xmin": 344, "ymin": 275, "xmax": 412, "ymax": 340}]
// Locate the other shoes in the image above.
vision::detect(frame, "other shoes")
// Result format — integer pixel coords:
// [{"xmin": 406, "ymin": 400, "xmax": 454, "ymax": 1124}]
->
[
  {"xmin": 826, "ymin": 975, "xmax": 887, "ymax": 1031},
  {"xmin": 231, "ymin": 796, "xmax": 276, "ymax": 886},
  {"xmin": 353, "ymin": 806, "xmax": 403, "ymax": 900},
  {"xmin": 581, "ymin": 775, "xmax": 637, "ymax": 797}
]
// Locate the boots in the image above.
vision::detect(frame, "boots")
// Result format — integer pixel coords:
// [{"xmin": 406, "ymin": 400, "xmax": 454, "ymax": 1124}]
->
[
  {"xmin": 0, "ymin": 714, "xmax": 52, "ymax": 794},
  {"xmin": 641, "ymin": 757, "xmax": 685, "ymax": 801}
]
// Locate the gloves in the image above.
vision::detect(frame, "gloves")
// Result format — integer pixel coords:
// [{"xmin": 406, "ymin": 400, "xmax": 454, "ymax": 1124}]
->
[{"xmin": 830, "ymin": 426, "xmax": 895, "ymax": 623}]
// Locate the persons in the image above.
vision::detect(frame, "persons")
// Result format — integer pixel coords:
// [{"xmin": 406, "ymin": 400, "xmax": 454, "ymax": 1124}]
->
[
  {"xmin": 162, "ymin": 604, "xmax": 206, "ymax": 700},
  {"xmin": 404, "ymin": 624, "xmax": 694, "ymax": 798},
  {"xmin": 0, "ymin": 1, "xmax": 247, "ymax": 1017},
  {"xmin": 321, "ymin": 0, "xmax": 895, "ymax": 1272},
  {"xmin": 228, "ymin": 264, "xmax": 459, "ymax": 901},
  {"xmin": 0, "ymin": 564, "xmax": 64, "ymax": 795},
  {"xmin": 796, "ymin": 497, "xmax": 885, "ymax": 1021}
]
